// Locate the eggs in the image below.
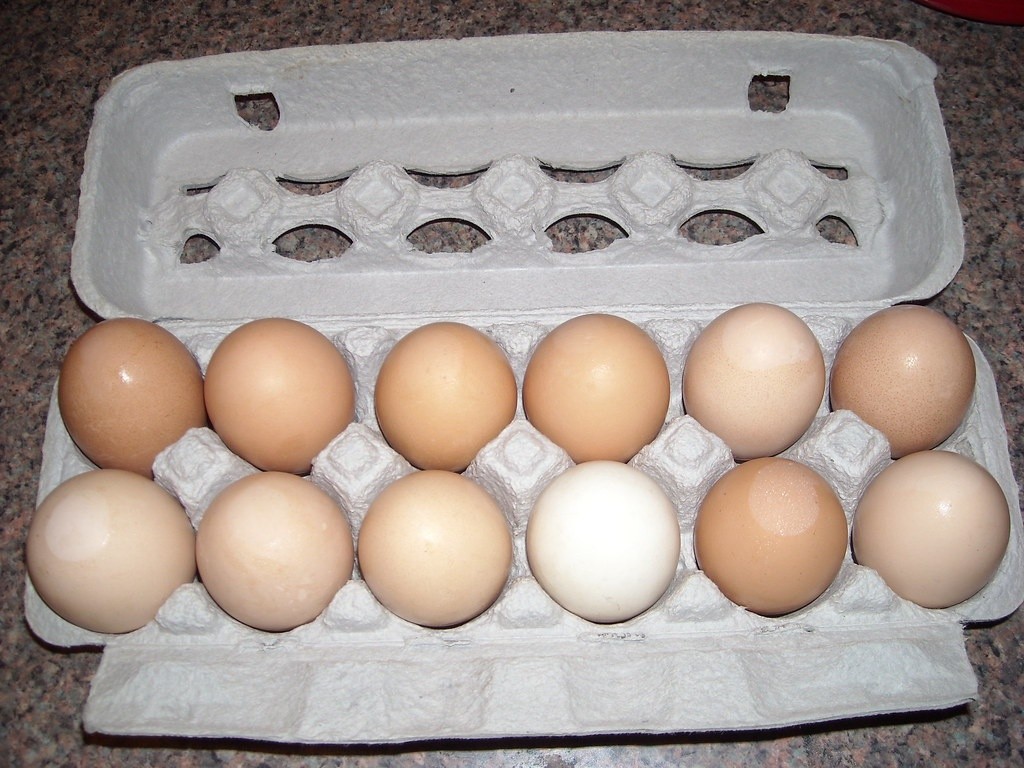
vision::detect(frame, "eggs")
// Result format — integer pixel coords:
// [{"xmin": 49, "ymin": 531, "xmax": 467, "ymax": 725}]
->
[
  {"xmin": 195, "ymin": 471, "xmax": 353, "ymax": 632},
  {"xmin": 204, "ymin": 318, "xmax": 355, "ymax": 476},
  {"xmin": 24, "ymin": 469, "xmax": 196, "ymax": 635},
  {"xmin": 356, "ymin": 469, "xmax": 512, "ymax": 627},
  {"xmin": 852, "ymin": 450, "xmax": 1010, "ymax": 609},
  {"xmin": 522, "ymin": 313, "xmax": 669, "ymax": 467},
  {"xmin": 57, "ymin": 318, "xmax": 208, "ymax": 479},
  {"xmin": 829, "ymin": 305, "xmax": 975, "ymax": 459},
  {"xmin": 681, "ymin": 302, "xmax": 826, "ymax": 461},
  {"xmin": 526, "ymin": 460, "xmax": 680, "ymax": 625},
  {"xmin": 374, "ymin": 321, "xmax": 518, "ymax": 473},
  {"xmin": 693, "ymin": 457, "xmax": 848, "ymax": 616}
]
